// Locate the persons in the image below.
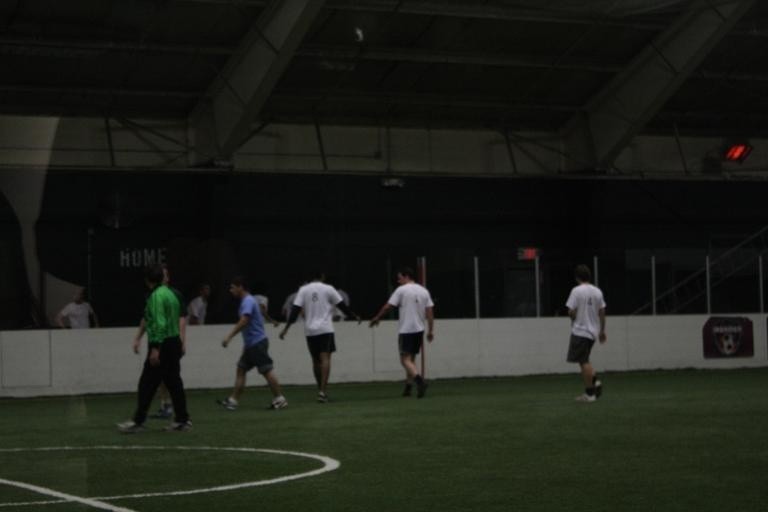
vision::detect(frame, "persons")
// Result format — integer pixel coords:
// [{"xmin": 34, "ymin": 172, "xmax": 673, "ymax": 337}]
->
[
  {"xmin": 115, "ymin": 263, "xmax": 192, "ymax": 432},
  {"xmin": 56, "ymin": 292, "xmax": 99, "ymax": 329},
  {"xmin": 217, "ymin": 276, "xmax": 288, "ymax": 410},
  {"xmin": 278, "ymin": 271, "xmax": 362, "ymax": 403},
  {"xmin": 186, "ymin": 284, "xmax": 211, "ymax": 325},
  {"xmin": 369, "ymin": 266, "xmax": 435, "ymax": 398},
  {"xmin": 565, "ymin": 264, "xmax": 607, "ymax": 402},
  {"xmin": 132, "ymin": 267, "xmax": 189, "ymax": 419},
  {"xmin": 254, "ymin": 288, "xmax": 350, "ymax": 327}
]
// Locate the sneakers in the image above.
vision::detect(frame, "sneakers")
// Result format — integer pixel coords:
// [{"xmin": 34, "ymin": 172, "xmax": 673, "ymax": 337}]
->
[
  {"xmin": 156, "ymin": 400, "xmax": 174, "ymax": 418},
  {"xmin": 116, "ymin": 419, "xmax": 146, "ymax": 434},
  {"xmin": 415, "ymin": 374, "xmax": 429, "ymax": 399},
  {"xmin": 402, "ymin": 379, "xmax": 413, "ymax": 398},
  {"xmin": 316, "ymin": 390, "xmax": 329, "ymax": 403},
  {"xmin": 215, "ymin": 395, "xmax": 241, "ymax": 413},
  {"xmin": 262, "ymin": 394, "xmax": 290, "ymax": 412},
  {"xmin": 160, "ymin": 419, "xmax": 193, "ymax": 435},
  {"xmin": 573, "ymin": 374, "xmax": 605, "ymax": 404}
]
